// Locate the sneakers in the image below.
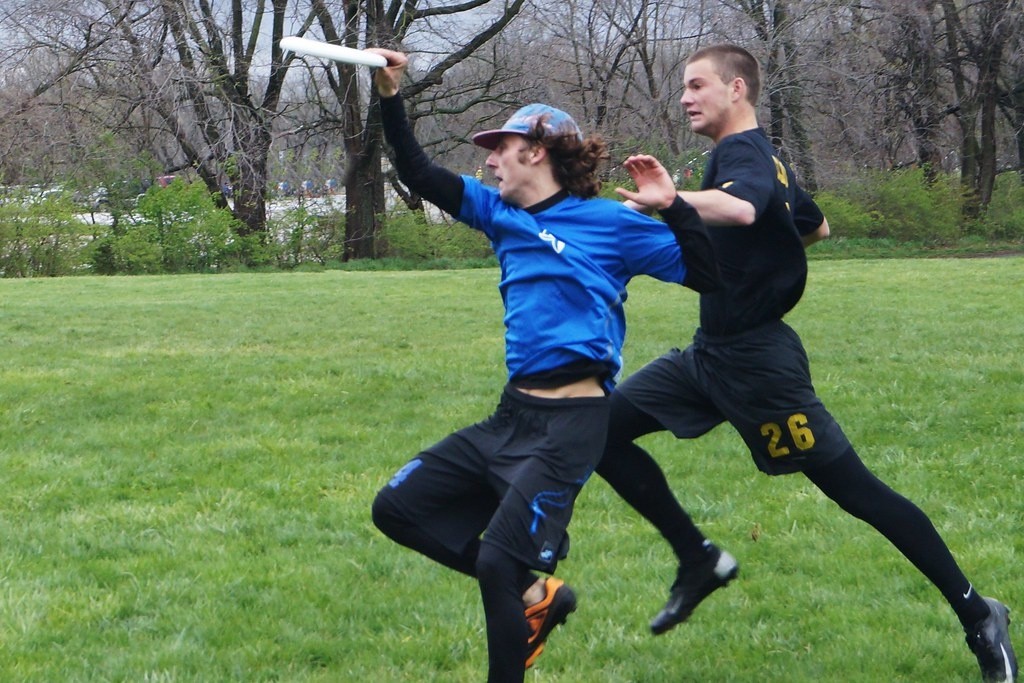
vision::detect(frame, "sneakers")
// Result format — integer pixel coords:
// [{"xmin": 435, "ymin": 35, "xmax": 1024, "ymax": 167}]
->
[
  {"xmin": 650, "ymin": 538, "xmax": 740, "ymax": 634},
  {"xmin": 523, "ymin": 577, "xmax": 577, "ymax": 669},
  {"xmin": 966, "ymin": 598, "xmax": 1019, "ymax": 683}
]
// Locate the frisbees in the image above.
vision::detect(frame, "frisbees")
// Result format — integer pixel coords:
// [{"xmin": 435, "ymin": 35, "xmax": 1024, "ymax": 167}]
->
[{"xmin": 276, "ymin": 34, "xmax": 388, "ymax": 69}]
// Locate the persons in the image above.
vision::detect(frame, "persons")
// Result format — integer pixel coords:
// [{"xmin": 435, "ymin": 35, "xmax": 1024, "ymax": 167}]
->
[
  {"xmin": 359, "ymin": 48, "xmax": 726, "ymax": 683},
  {"xmin": 594, "ymin": 45, "xmax": 1018, "ymax": 683}
]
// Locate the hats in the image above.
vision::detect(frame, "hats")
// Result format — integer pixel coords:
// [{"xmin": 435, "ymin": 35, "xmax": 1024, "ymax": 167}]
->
[{"xmin": 472, "ymin": 103, "xmax": 583, "ymax": 150}]
